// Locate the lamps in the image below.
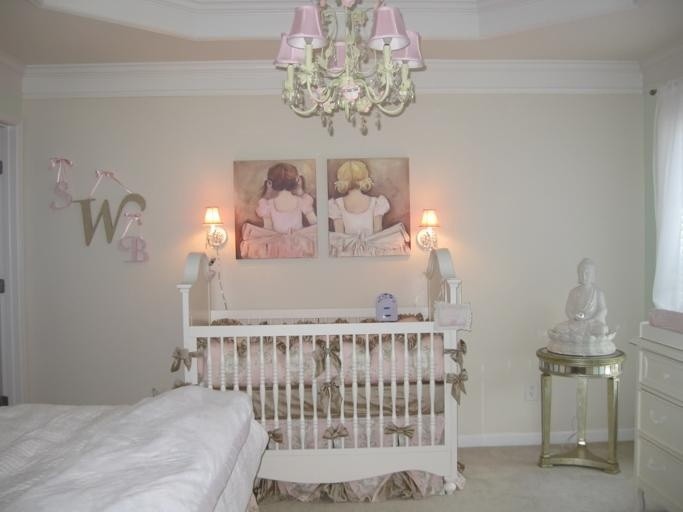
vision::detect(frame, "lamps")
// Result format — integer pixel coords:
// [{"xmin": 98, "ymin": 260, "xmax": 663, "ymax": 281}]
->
[
  {"xmin": 203, "ymin": 205, "xmax": 227, "ymax": 246},
  {"xmin": 273, "ymin": 0, "xmax": 427, "ymax": 138},
  {"xmin": 416, "ymin": 208, "xmax": 439, "ymax": 248}
]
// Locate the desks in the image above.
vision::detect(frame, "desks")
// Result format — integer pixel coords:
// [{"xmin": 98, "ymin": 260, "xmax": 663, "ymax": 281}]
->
[{"xmin": 536, "ymin": 347, "xmax": 628, "ymax": 475}]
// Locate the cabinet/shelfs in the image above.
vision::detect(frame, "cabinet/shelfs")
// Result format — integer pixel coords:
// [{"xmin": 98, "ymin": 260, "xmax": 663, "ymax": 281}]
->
[{"xmin": 630, "ymin": 321, "xmax": 683, "ymax": 511}]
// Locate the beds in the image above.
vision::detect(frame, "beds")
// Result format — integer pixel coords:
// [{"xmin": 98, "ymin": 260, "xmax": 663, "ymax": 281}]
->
[
  {"xmin": 0, "ymin": 385, "xmax": 270, "ymax": 512},
  {"xmin": 173, "ymin": 249, "xmax": 470, "ymax": 501}
]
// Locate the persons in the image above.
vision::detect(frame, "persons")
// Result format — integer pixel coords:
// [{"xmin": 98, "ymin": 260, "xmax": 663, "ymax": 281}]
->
[
  {"xmin": 240, "ymin": 163, "xmax": 316, "ymax": 258},
  {"xmin": 328, "ymin": 160, "xmax": 410, "ymax": 256},
  {"xmin": 552, "ymin": 258, "xmax": 608, "ymax": 334}
]
